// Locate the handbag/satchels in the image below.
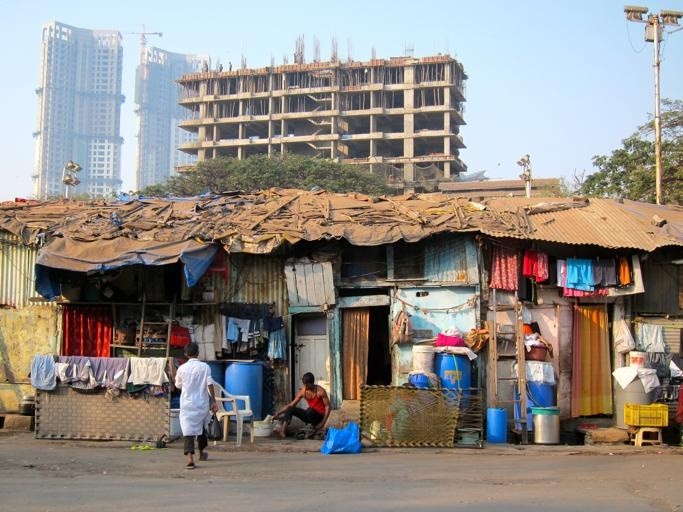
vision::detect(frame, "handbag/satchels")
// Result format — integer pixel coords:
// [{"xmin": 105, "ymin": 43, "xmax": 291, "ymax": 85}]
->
[{"xmin": 209, "ymin": 416, "xmax": 221, "ymax": 440}]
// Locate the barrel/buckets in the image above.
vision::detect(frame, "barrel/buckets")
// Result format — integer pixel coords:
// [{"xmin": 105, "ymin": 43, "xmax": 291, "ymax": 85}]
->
[
  {"xmin": 407, "ymin": 375, "xmax": 431, "ymax": 388},
  {"xmin": 486, "ymin": 407, "xmax": 508, "ymax": 443},
  {"xmin": 514, "ymin": 380, "xmax": 558, "ymax": 431},
  {"xmin": 614, "ymin": 368, "xmax": 657, "ymax": 430},
  {"xmin": 434, "ymin": 353, "xmax": 473, "ymax": 411},
  {"xmin": 223, "ymin": 362, "xmax": 264, "ymax": 421},
  {"xmin": 411, "ymin": 344, "xmax": 434, "ymax": 374},
  {"xmin": 629, "ymin": 351, "xmax": 646, "ymax": 369},
  {"xmin": 202, "ymin": 361, "xmax": 223, "ymax": 386},
  {"xmin": 252, "ymin": 422, "xmax": 273, "ymax": 437}
]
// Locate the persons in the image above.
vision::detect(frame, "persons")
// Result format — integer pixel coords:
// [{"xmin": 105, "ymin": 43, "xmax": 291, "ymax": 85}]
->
[
  {"xmin": 269, "ymin": 372, "xmax": 330, "ymax": 439},
  {"xmin": 385, "ymin": 382, "xmax": 436, "ymax": 448},
  {"xmin": 175, "ymin": 342, "xmax": 220, "ymax": 469}
]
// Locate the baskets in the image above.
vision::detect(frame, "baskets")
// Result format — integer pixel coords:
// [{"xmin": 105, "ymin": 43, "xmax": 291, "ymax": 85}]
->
[{"xmin": 624, "ymin": 403, "xmax": 669, "ymax": 427}]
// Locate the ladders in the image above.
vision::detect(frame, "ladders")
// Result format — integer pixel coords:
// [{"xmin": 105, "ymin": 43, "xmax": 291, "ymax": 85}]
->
[
  {"xmin": 489, "ymin": 288, "xmax": 528, "ymax": 445},
  {"xmin": 138, "ymin": 292, "xmax": 175, "ymax": 357}
]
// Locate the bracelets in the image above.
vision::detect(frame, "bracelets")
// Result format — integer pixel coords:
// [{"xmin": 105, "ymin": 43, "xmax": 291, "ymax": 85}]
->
[{"xmin": 389, "ymin": 436, "xmax": 393, "ymax": 440}]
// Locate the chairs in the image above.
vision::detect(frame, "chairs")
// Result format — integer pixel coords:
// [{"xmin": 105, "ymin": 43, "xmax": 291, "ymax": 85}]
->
[{"xmin": 207, "ymin": 380, "xmax": 255, "ymax": 448}]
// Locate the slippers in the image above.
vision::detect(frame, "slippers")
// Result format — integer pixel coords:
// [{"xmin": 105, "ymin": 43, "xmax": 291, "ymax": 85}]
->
[
  {"xmin": 297, "ymin": 431, "xmax": 305, "ymax": 439},
  {"xmin": 184, "ymin": 464, "xmax": 194, "ymax": 468}
]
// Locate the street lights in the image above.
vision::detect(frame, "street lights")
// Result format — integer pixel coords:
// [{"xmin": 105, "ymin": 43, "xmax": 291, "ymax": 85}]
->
[
  {"xmin": 518, "ymin": 154, "xmax": 535, "ymax": 198},
  {"xmin": 61, "ymin": 160, "xmax": 83, "ymax": 199},
  {"xmin": 623, "ymin": 4, "xmax": 682, "ymax": 207}
]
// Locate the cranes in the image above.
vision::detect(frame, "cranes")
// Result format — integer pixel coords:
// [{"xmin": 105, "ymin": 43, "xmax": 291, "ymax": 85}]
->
[{"xmin": 122, "ymin": 24, "xmax": 164, "ymax": 47}]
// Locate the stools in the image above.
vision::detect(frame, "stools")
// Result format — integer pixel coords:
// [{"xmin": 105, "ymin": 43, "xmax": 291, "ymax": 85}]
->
[{"xmin": 629, "ymin": 426, "xmax": 663, "ymax": 447}]
